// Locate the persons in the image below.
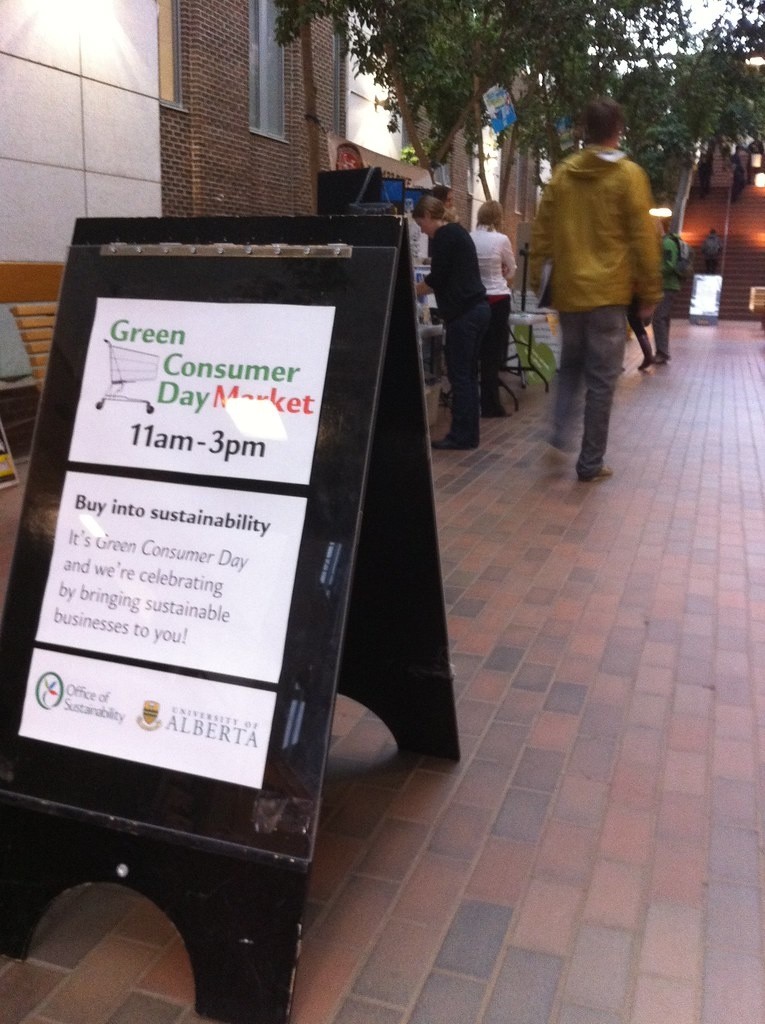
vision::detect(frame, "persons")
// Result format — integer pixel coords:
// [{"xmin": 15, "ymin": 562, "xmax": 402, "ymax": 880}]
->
[
  {"xmin": 412, "ymin": 184, "xmax": 491, "ymax": 452},
  {"xmin": 469, "ymin": 201, "xmax": 517, "ymax": 418},
  {"xmin": 627, "ymin": 220, "xmax": 682, "ymax": 371},
  {"xmin": 698, "ymin": 120, "xmax": 764, "ymax": 205},
  {"xmin": 701, "ymin": 228, "xmax": 724, "ymax": 273},
  {"xmin": 529, "ymin": 96, "xmax": 665, "ymax": 484}
]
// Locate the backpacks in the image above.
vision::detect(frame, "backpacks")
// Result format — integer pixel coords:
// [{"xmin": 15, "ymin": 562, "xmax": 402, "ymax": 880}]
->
[{"xmin": 663, "ymin": 236, "xmax": 695, "ymax": 275}]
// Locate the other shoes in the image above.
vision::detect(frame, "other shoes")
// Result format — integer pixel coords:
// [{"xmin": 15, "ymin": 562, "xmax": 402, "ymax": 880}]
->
[
  {"xmin": 638, "ymin": 354, "xmax": 655, "ymax": 369},
  {"xmin": 588, "ymin": 466, "xmax": 613, "ymax": 483},
  {"xmin": 431, "ymin": 434, "xmax": 478, "ymax": 449},
  {"xmin": 482, "ymin": 409, "xmax": 512, "ymax": 417},
  {"xmin": 654, "ymin": 353, "xmax": 666, "ymax": 364}
]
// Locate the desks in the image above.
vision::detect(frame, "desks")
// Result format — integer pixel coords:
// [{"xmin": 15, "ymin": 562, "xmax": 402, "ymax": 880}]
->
[{"xmin": 506, "ymin": 312, "xmax": 560, "ymax": 393}]
